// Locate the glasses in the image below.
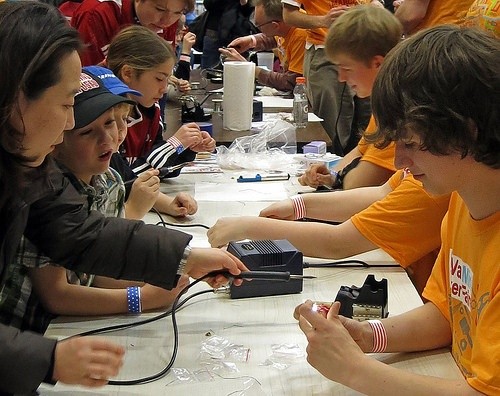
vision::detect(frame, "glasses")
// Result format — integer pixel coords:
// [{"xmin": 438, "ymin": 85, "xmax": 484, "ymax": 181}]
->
[{"xmin": 254, "ymin": 20, "xmax": 280, "ymax": 30}]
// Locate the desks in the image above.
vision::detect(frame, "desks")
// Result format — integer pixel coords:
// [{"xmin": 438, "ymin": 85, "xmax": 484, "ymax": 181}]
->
[{"xmin": 37, "ymin": 69, "xmax": 469, "ymax": 396}]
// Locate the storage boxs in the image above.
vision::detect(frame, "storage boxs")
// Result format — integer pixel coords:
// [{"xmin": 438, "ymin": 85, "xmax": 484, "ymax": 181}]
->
[
  {"xmin": 318, "ymin": 157, "xmax": 343, "ymax": 169},
  {"xmin": 303, "ymin": 140, "xmax": 326, "ymax": 155}
]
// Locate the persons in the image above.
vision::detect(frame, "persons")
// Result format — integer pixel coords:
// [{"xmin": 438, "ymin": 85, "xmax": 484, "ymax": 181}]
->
[
  {"xmin": 293, "ymin": 27, "xmax": 500, "ymax": 396},
  {"xmin": 0, "ymin": 0, "xmax": 204, "ymax": 335},
  {"xmin": 200, "ymin": 0, "xmax": 473, "ymax": 183},
  {"xmin": 0, "ymin": 0, "xmax": 252, "ymax": 396},
  {"xmin": 207, "ymin": 0, "xmax": 500, "ymax": 304}
]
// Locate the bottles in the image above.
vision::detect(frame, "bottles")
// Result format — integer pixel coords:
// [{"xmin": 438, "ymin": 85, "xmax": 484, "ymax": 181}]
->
[{"xmin": 293, "ymin": 76, "xmax": 309, "ymax": 123}]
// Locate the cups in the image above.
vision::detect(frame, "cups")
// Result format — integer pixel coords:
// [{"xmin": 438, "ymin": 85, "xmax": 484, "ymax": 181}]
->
[{"xmin": 256, "ymin": 52, "xmax": 274, "ymax": 72}]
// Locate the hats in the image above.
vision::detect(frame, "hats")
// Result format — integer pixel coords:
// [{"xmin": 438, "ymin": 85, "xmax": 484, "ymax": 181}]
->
[
  {"xmin": 73, "ymin": 69, "xmax": 139, "ymax": 129},
  {"xmin": 83, "ymin": 66, "xmax": 143, "ymax": 98}
]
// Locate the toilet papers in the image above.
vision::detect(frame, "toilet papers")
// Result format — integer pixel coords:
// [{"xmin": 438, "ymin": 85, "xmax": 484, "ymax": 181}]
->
[{"xmin": 221, "ymin": 60, "xmax": 257, "ymax": 132}]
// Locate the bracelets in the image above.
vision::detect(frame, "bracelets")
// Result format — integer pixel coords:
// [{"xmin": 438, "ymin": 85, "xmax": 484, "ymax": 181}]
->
[
  {"xmin": 127, "ymin": 286, "xmax": 142, "ymax": 313},
  {"xmin": 168, "ymin": 135, "xmax": 186, "ymax": 154},
  {"xmin": 367, "ymin": 320, "xmax": 387, "ymax": 353},
  {"xmin": 176, "ymin": 245, "xmax": 191, "ymax": 275},
  {"xmin": 180, "ymin": 55, "xmax": 191, "ymax": 62},
  {"xmin": 290, "ymin": 194, "xmax": 306, "ymax": 220},
  {"xmin": 250, "ymin": 35, "xmax": 256, "ymax": 48}
]
